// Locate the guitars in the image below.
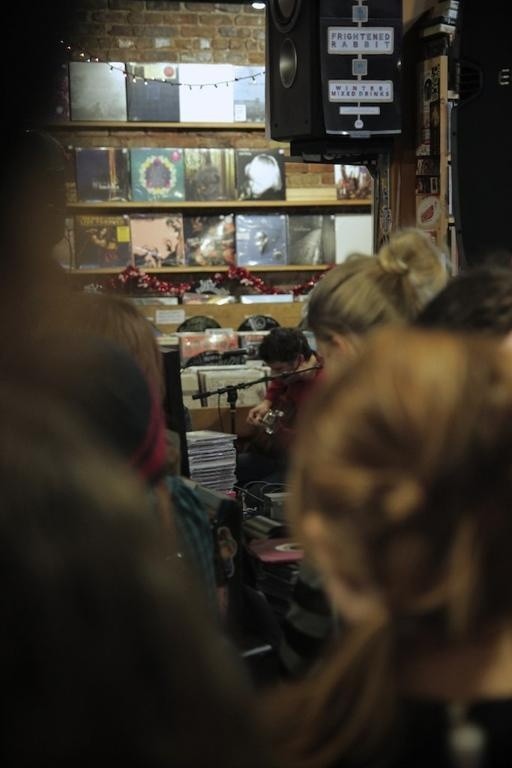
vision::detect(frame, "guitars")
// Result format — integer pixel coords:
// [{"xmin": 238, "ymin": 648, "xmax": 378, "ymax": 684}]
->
[{"xmin": 251, "ymin": 399, "xmax": 296, "ymax": 444}]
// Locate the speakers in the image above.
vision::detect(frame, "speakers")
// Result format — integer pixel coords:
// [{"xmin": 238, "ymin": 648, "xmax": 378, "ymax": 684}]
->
[
  {"xmin": 266, "ymin": 0, "xmax": 406, "ymax": 142},
  {"xmin": 456, "ymin": 0, "xmax": 512, "ymax": 262}
]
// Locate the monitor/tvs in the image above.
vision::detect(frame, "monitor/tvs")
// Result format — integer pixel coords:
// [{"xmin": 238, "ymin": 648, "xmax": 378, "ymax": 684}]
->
[{"xmin": 160, "ymin": 347, "xmax": 190, "ymax": 479}]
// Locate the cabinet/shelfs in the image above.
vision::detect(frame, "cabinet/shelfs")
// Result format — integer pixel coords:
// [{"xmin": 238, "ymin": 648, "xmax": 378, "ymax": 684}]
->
[{"xmin": 47, "ymin": 121, "xmax": 383, "ymax": 354}]
